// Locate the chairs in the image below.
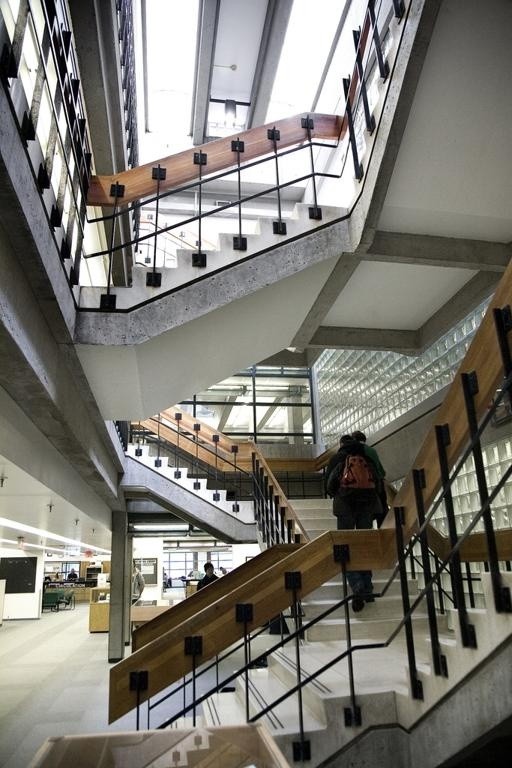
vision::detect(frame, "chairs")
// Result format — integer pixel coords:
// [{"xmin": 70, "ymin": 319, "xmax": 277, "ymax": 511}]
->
[
  {"xmin": 49, "ymin": 583, "xmax": 75, "ymax": 588},
  {"xmin": 42, "ymin": 585, "xmax": 75, "ymax": 613}
]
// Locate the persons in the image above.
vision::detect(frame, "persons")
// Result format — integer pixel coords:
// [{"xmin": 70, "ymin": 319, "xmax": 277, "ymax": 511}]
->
[
  {"xmin": 197, "ymin": 562, "xmax": 219, "ymax": 591},
  {"xmin": 324, "ymin": 430, "xmax": 388, "ymax": 612},
  {"xmin": 68, "ymin": 568, "xmax": 77, "ymax": 578},
  {"xmin": 132, "ymin": 559, "xmax": 145, "ymax": 604}
]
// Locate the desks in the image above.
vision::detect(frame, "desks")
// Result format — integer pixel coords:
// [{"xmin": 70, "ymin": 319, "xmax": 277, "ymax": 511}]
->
[
  {"xmin": 44, "ymin": 580, "xmax": 98, "ymax": 588},
  {"xmin": 26, "ymin": 722, "xmax": 292, "ymax": 768}
]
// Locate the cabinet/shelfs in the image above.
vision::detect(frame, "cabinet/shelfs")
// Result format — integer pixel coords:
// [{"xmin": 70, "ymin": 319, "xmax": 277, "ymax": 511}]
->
[{"xmin": 87, "ymin": 566, "xmax": 102, "ymax": 579}]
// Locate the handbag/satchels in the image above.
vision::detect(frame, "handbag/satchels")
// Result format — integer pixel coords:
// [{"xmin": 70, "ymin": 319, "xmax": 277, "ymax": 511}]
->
[{"xmin": 340, "ymin": 455, "xmax": 376, "ymax": 495}]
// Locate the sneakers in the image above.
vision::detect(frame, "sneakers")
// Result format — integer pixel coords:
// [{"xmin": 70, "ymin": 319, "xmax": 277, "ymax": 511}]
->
[{"xmin": 351, "ymin": 583, "xmax": 375, "ymax": 612}]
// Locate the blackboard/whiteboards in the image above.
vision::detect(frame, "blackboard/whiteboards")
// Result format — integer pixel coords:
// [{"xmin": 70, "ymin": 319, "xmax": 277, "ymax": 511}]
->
[{"xmin": 0, "ymin": 557, "xmax": 37, "ymax": 593}]
